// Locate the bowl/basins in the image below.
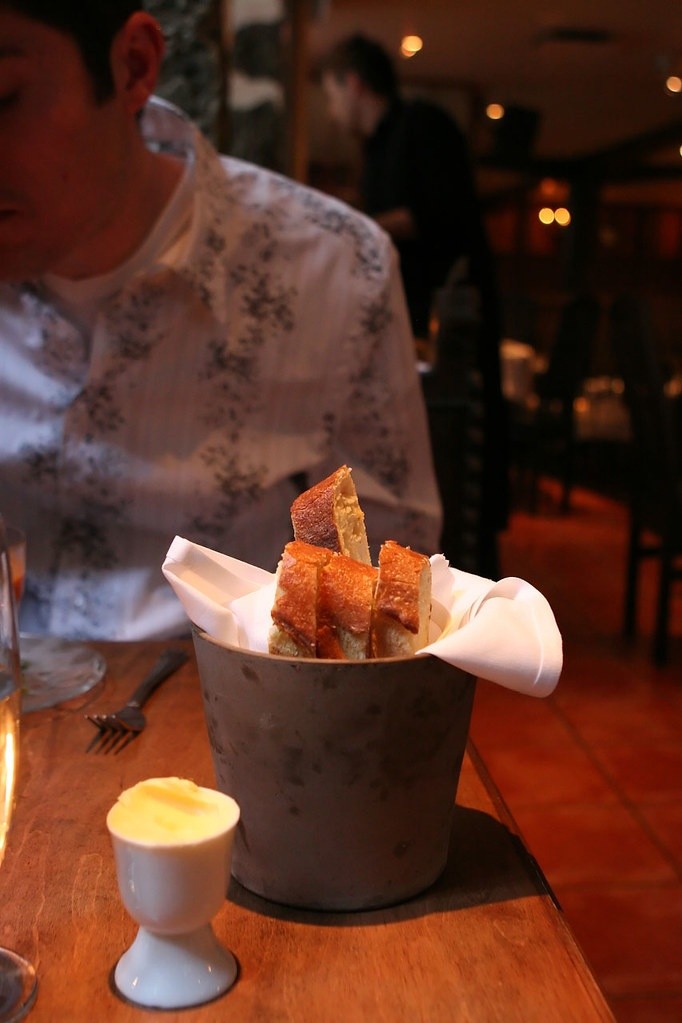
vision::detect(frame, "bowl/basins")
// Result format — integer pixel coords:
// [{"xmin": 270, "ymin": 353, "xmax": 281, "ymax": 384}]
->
[{"xmin": 188, "ymin": 622, "xmax": 478, "ymax": 914}]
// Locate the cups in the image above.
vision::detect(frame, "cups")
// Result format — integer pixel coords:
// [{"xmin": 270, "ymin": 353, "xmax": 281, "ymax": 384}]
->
[{"xmin": 107, "ymin": 788, "xmax": 239, "ymax": 1008}]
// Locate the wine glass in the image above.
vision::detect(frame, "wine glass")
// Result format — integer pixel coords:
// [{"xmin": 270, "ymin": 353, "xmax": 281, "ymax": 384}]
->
[{"xmin": 0, "ymin": 550, "xmax": 38, "ymax": 1023}]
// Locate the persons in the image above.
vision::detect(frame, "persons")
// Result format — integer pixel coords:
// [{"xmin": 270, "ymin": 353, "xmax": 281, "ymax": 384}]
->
[
  {"xmin": 0, "ymin": 0, "xmax": 443, "ymax": 643},
  {"xmin": 305, "ymin": 31, "xmax": 509, "ymax": 580}
]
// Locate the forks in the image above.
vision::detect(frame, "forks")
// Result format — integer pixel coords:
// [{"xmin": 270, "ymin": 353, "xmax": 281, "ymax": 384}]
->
[{"xmin": 84, "ymin": 648, "xmax": 189, "ymax": 732}]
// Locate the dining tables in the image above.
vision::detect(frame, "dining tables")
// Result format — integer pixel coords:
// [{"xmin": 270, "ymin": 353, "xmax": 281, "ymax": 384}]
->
[{"xmin": 0, "ymin": 636, "xmax": 621, "ymax": 1023}]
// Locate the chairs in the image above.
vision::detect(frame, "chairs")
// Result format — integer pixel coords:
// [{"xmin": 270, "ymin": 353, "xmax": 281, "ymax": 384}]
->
[
  {"xmin": 609, "ymin": 289, "xmax": 682, "ymax": 666},
  {"xmin": 502, "ymin": 293, "xmax": 597, "ymax": 516},
  {"xmin": 418, "ymin": 247, "xmax": 499, "ymax": 582}
]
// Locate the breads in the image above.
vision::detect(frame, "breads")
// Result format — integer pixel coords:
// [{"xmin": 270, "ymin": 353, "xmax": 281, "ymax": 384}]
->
[
  {"xmin": 369, "ymin": 540, "xmax": 433, "ymax": 658},
  {"xmin": 291, "ymin": 464, "xmax": 371, "ymax": 566},
  {"xmin": 266, "ymin": 542, "xmax": 333, "ymax": 660},
  {"xmin": 314, "ymin": 554, "xmax": 380, "ymax": 660}
]
